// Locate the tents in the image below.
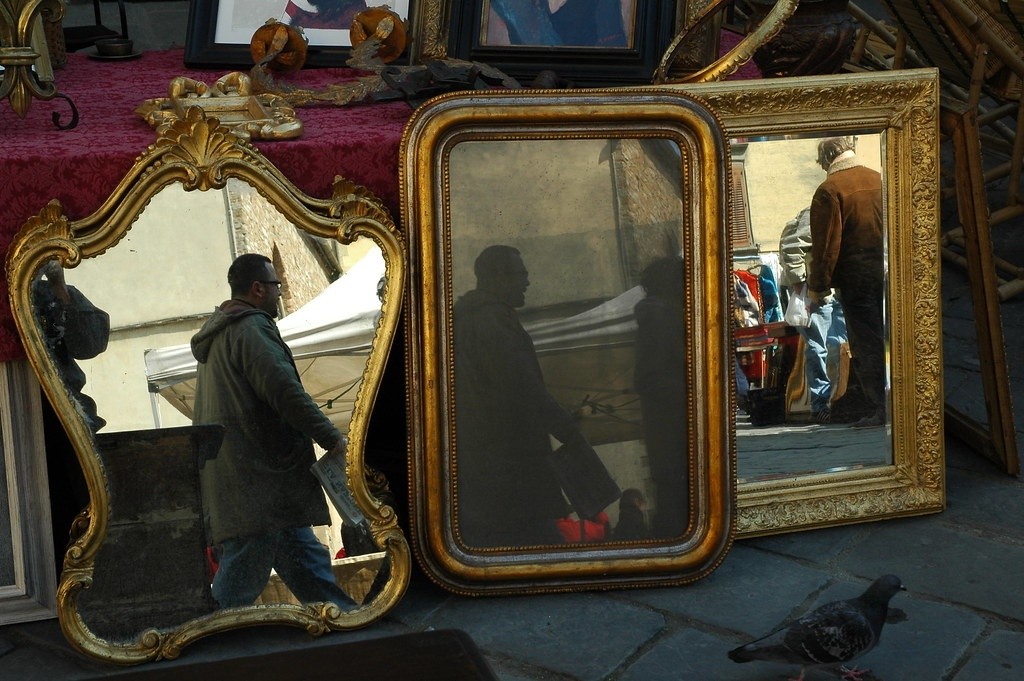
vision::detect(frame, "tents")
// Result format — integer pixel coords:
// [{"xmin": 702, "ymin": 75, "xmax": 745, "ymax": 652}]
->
[
  {"xmin": 522, "ymin": 249, "xmax": 686, "ymax": 356},
  {"xmin": 143, "ymin": 246, "xmax": 385, "ymax": 443}
]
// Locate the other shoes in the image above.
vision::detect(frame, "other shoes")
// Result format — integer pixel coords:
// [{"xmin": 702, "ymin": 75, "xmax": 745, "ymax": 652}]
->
[
  {"xmin": 806, "ymin": 408, "xmax": 831, "ymax": 425},
  {"xmin": 852, "ymin": 414, "xmax": 886, "ymax": 430}
]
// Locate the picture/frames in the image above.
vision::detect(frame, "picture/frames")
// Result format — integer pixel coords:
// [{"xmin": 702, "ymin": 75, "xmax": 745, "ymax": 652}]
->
[
  {"xmin": 406, "ymin": 0, "xmax": 724, "ymax": 93},
  {"xmin": 182, "ymin": 0, "xmax": 443, "ymax": 73}
]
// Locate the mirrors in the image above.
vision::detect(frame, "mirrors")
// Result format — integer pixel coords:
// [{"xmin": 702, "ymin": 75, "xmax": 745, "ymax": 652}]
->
[
  {"xmin": 655, "ymin": 64, "xmax": 946, "ymax": 545},
  {"xmin": 397, "ymin": 87, "xmax": 735, "ymax": 604},
  {"xmin": 0, "ymin": 105, "xmax": 415, "ymax": 664}
]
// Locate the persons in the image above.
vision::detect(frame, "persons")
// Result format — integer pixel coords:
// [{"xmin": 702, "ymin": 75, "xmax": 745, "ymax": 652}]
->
[
  {"xmin": 188, "ymin": 254, "xmax": 361, "ymax": 615},
  {"xmin": 780, "ymin": 136, "xmax": 888, "ymax": 427},
  {"xmin": 31, "ymin": 260, "xmax": 110, "ymax": 431},
  {"xmin": 452, "ymin": 244, "xmax": 685, "ymax": 550}
]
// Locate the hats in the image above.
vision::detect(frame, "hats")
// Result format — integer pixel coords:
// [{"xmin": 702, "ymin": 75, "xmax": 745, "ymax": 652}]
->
[{"xmin": 76, "ymin": 392, "xmax": 106, "ymax": 434}]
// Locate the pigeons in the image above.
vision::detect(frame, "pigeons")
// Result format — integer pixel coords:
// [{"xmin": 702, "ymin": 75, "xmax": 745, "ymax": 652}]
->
[{"xmin": 727, "ymin": 575, "xmax": 908, "ymax": 681}]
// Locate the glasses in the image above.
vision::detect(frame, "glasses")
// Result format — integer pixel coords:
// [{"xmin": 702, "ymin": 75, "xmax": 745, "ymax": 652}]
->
[
  {"xmin": 506, "ymin": 269, "xmax": 529, "ymax": 283},
  {"xmin": 260, "ymin": 279, "xmax": 283, "ymax": 290},
  {"xmin": 377, "ymin": 288, "xmax": 386, "ymax": 298}
]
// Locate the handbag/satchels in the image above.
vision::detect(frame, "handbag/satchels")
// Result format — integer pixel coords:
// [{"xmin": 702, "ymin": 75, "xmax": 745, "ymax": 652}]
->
[
  {"xmin": 782, "ymin": 282, "xmax": 815, "ymax": 328},
  {"xmin": 746, "ymin": 384, "xmax": 786, "ymax": 426},
  {"xmin": 831, "ymin": 342, "xmax": 853, "ymax": 402},
  {"xmin": 732, "ymin": 265, "xmax": 779, "ymax": 328}
]
